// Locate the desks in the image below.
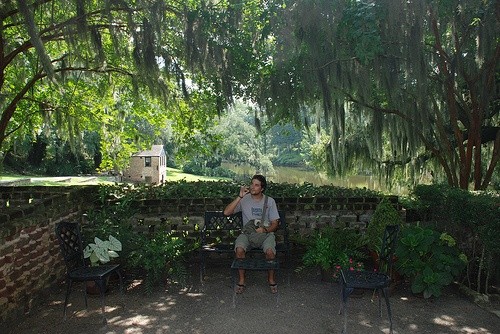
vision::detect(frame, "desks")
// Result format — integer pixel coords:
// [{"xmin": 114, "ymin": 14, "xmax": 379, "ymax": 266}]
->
[{"xmin": 230, "ymin": 258, "xmax": 283, "ymax": 308}]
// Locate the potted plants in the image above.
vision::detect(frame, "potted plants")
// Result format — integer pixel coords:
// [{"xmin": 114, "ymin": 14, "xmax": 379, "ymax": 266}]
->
[{"xmin": 296, "ymin": 230, "xmax": 367, "ymax": 283}]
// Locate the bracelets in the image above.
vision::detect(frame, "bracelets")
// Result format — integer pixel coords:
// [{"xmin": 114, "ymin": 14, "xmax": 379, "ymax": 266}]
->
[
  {"xmin": 264, "ymin": 228, "xmax": 268, "ymax": 233},
  {"xmin": 238, "ymin": 195, "xmax": 243, "ymax": 199}
]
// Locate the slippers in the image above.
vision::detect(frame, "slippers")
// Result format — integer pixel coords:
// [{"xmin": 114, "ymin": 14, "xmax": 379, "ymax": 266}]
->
[
  {"xmin": 269, "ymin": 283, "xmax": 278, "ymax": 294},
  {"xmin": 236, "ymin": 284, "xmax": 244, "ymax": 294}
]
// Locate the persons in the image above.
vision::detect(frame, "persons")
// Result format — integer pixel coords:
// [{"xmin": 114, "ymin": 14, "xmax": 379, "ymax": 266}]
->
[{"xmin": 224, "ymin": 175, "xmax": 279, "ymax": 295}]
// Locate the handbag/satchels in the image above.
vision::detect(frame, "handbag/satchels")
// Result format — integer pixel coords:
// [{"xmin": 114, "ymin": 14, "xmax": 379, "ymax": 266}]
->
[{"xmin": 242, "ymin": 218, "xmax": 268, "ymax": 247}]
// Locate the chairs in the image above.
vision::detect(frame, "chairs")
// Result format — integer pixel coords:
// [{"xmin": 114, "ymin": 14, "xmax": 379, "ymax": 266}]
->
[
  {"xmin": 339, "ymin": 225, "xmax": 399, "ymax": 334},
  {"xmin": 56, "ymin": 221, "xmax": 127, "ymax": 327}
]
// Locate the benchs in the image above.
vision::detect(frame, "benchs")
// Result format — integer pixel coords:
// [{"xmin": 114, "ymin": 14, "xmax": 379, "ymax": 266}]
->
[{"xmin": 189, "ymin": 210, "xmax": 290, "ymax": 294}]
